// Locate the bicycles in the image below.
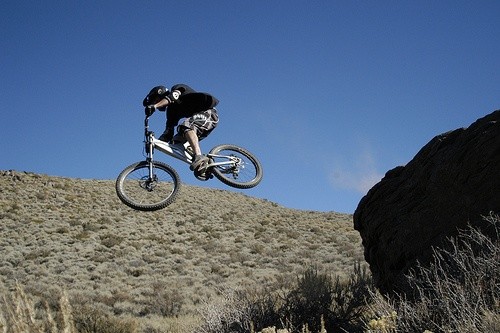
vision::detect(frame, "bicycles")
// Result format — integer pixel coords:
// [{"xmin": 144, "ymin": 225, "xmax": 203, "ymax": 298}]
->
[{"xmin": 115, "ymin": 107, "xmax": 264, "ymax": 212}]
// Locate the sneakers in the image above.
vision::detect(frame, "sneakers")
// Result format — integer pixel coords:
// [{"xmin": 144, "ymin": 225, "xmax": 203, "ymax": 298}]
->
[{"xmin": 189, "ymin": 154, "xmax": 210, "ymax": 171}]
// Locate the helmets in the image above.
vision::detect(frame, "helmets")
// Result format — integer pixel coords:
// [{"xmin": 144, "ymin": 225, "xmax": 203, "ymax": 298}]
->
[{"xmin": 143, "ymin": 85, "xmax": 169, "ymax": 112}]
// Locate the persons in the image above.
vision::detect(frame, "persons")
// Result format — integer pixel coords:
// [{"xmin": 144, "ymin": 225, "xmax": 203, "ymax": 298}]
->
[{"xmin": 145, "ymin": 83, "xmax": 219, "ymax": 172}]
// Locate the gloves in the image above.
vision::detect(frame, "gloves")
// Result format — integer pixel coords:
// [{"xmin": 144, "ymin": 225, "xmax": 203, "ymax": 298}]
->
[{"xmin": 145, "ymin": 105, "xmax": 156, "ymax": 116}]
